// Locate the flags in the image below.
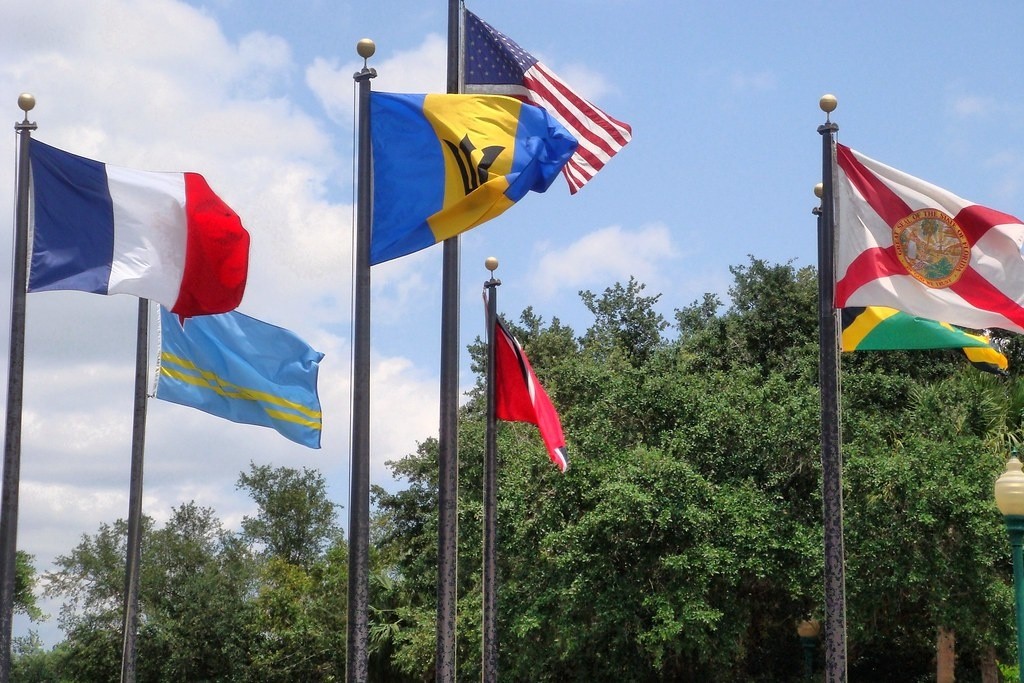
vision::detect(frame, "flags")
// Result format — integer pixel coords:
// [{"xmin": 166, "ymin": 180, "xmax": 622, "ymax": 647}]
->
[
  {"xmin": 840, "ymin": 307, "xmax": 1009, "ymax": 375},
  {"xmin": 370, "ymin": 91, "xmax": 578, "ymax": 269},
  {"xmin": 27, "ymin": 133, "xmax": 251, "ymax": 318},
  {"xmin": 482, "ymin": 290, "xmax": 570, "ymax": 474},
  {"xmin": 146, "ymin": 292, "xmax": 325, "ymax": 449},
  {"xmin": 831, "ymin": 141, "xmax": 1024, "ymax": 334},
  {"xmin": 460, "ymin": 4, "xmax": 632, "ymax": 195}
]
